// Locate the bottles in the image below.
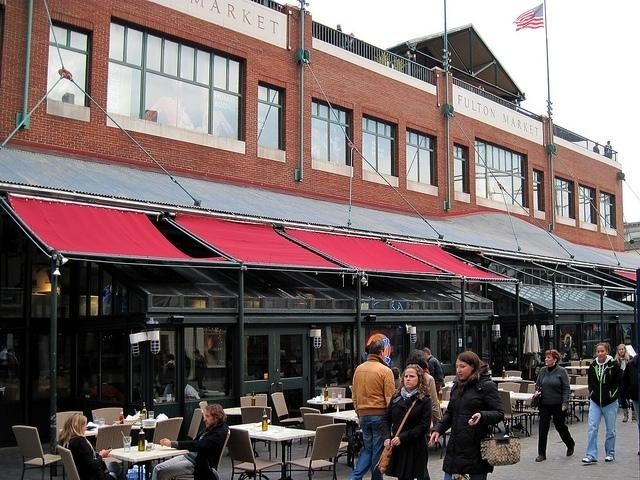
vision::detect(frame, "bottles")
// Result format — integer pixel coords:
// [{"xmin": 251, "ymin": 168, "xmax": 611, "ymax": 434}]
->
[
  {"xmin": 502, "ymin": 366, "xmax": 506, "ymax": 379},
  {"xmin": 324, "ymin": 384, "xmax": 328, "ymax": 401},
  {"xmin": 142, "ymin": 401, "xmax": 147, "ymax": 419},
  {"xmin": 138, "ymin": 427, "xmax": 145, "ymax": 451},
  {"xmin": 119, "ymin": 412, "xmax": 124, "ymax": 424},
  {"xmin": 579, "ymin": 358, "xmax": 581, "ymax": 367},
  {"xmin": 262, "ymin": 409, "xmax": 268, "ymax": 431},
  {"xmin": 251, "ymin": 391, "xmax": 256, "ymax": 406}
]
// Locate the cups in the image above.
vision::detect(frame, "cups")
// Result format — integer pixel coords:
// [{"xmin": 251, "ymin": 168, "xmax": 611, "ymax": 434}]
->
[
  {"xmin": 332, "ymin": 392, "xmax": 337, "ymax": 403},
  {"xmin": 338, "ymin": 393, "xmax": 343, "ymax": 400},
  {"xmin": 123, "ymin": 436, "xmax": 131, "ymax": 452},
  {"xmin": 149, "ymin": 411, "xmax": 154, "ymax": 420},
  {"xmin": 99, "ymin": 418, "xmax": 105, "ymax": 426}
]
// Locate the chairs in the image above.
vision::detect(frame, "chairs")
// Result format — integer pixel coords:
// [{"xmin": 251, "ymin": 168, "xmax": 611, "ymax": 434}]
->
[
  {"xmin": 525, "ymin": 385, "xmax": 541, "ymax": 424},
  {"xmin": 91, "ymin": 407, "xmax": 124, "ymax": 426},
  {"xmin": 96, "ymin": 424, "xmax": 132, "ymax": 452},
  {"xmin": 240, "ymin": 395, "xmax": 265, "ymax": 406},
  {"xmin": 12, "ymin": 425, "xmax": 66, "ymax": 480},
  {"xmin": 498, "ymin": 391, "xmax": 528, "ymax": 437},
  {"xmin": 300, "ymin": 406, "xmax": 320, "ymax": 421},
  {"xmin": 572, "ymin": 360, "xmax": 587, "ymax": 375},
  {"xmin": 241, "ymin": 406, "xmax": 271, "ymax": 424},
  {"xmin": 498, "ymin": 383, "xmax": 520, "ymax": 393},
  {"xmin": 321, "ymin": 388, "xmax": 345, "ymax": 411},
  {"xmin": 175, "ymin": 428, "xmax": 230, "ymax": 480},
  {"xmin": 505, "ymin": 371, "xmax": 521, "ymax": 377},
  {"xmin": 271, "ymin": 392, "xmax": 304, "ymax": 445},
  {"xmin": 55, "ymin": 411, "xmax": 83, "ymax": 442},
  {"xmin": 303, "ymin": 413, "xmax": 334, "ymax": 458},
  {"xmin": 152, "ymin": 417, "xmax": 183, "ymax": 445},
  {"xmin": 199, "ymin": 400, "xmax": 208, "ymax": 417},
  {"xmin": 57, "ymin": 445, "xmax": 82, "ymax": 480},
  {"xmin": 229, "ymin": 427, "xmax": 283, "ymax": 480},
  {"xmin": 572, "ymin": 376, "xmax": 588, "ymax": 397},
  {"xmin": 188, "ymin": 408, "xmax": 203, "ymax": 440}
]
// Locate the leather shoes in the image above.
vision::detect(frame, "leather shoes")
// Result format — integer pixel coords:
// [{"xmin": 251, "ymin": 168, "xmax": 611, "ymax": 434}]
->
[
  {"xmin": 536, "ymin": 455, "xmax": 547, "ymax": 462},
  {"xmin": 567, "ymin": 446, "xmax": 575, "ymax": 457}
]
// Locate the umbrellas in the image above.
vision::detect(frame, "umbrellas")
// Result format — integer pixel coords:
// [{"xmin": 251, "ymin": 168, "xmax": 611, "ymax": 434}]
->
[{"xmin": 524, "ymin": 303, "xmax": 540, "ymax": 379}]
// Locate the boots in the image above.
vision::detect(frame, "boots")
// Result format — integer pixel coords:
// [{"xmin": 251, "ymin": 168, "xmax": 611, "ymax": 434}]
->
[
  {"xmin": 622, "ymin": 408, "xmax": 630, "ymax": 422},
  {"xmin": 632, "ymin": 412, "xmax": 637, "ymax": 424}
]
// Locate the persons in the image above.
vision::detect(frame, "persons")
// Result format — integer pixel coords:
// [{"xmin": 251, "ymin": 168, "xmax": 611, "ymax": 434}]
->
[
  {"xmin": 160, "ymin": 354, "xmax": 177, "ymax": 396},
  {"xmin": 153, "ymin": 403, "xmax": 228, "ymax": 480},
  {"xmin": 615, "ymin": 344, "xmax": 638, "ymax": 422},
  {"xmin": 405, "ymin": 349, "xmax": 441, "ymax": 424},
  {"xmin": 8, "ymin": 349, "xmax": 16, "ymax": 366},
  {"xmin": 335, "ymin": 24, "xmax": 354, "ymax": 50},
  {"xmin": 347, "ymin": 341, "xmax": 395, "ymax": 480},
  {"xmin": 332, "ymin": 339, "xmax": 348, "ymax": 360},
  {"xmin": 581, "ymin": 343, "xmax": 619, "ymax": 464},
  {"xmin": 58, "ymin": 414, "xmax": 115, "ymax": 480},
  {"xmin": 623, "ymin": 343, "xmax": 640, "ymax": 455},
  {"xmin": 604, "ymin": 140, "xmax": 614, "ymax": 157},
  {"xmin": 427, "ymin": 352, "xmax": 506, "ymax": 480},
  {"xmin": 0, "ymin": 343, "xmax": 9, "ymax": 376},
  {"xmin": 423, "ymin": 348, "xmax": 445, "ymax": 401},
  {"xmin": 530, "ymin": 349, "xmax": 576, "ymax": 462},
  {"xmin": 193, "ymin": 349, "xmax": 207, "ymax": 388},
  {"xmin": 594, "ymin": 143, "xmax": 599, "ymax": 153},
  {"xmin": 92, "ymin": 373, "xmax": 122, "ymax": 399},
  {"xmin": 381, "ymin": 364, "xmax": 430, "ymax": 480}
]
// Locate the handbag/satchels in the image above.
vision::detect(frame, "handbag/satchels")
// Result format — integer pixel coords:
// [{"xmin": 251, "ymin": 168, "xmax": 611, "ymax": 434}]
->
[
  {"xmin": 372, "ymin": 399, "xmax": 419, "ymax": 474},
  {"xmin": 530, "ymin": 367, "xmax": 547, "ymax": 408},
  {"xmin": 479, "ymin": 409, "xmax": 522, "ymax": 466}
]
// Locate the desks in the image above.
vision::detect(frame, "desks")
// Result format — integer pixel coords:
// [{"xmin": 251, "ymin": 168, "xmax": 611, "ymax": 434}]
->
[
  {"xmin": 306, "ymin": 398, "xmax": 353, "ymax": 412},
  {"xmin": 84, "ymin": 425, "xmax": 156, "ymax": 436},
  {"xmin": 229, "ymin": 423, "xmax": 316, "ymax": 480},
  {"xmin": 491, "ymin": 376, "xmax": 522, "ymax": 382},
  {"xmin": 323, "ymin": 409, "xmax": 361, "ymax": 466},
  {"xmin": 223, "ymin": 407, "xmax": 241, "ymax": 416},
  {"xmin": 106, "ymin": 442, "xmax": 190, "ymax": 480},
  {"xmin": 513, "ymin": 392, "xmax": 534, "ymax": 412},
  {"xmin": 565, "ymin": 366, "xmax": 590, "ymax": 375},
  {"xmin": 569, "ymin": 384, "xmax": 588, "ymax": 421}
]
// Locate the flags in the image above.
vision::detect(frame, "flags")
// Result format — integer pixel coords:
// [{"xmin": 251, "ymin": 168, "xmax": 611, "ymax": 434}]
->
[{"xmin": 513, "ymin": 0, "xmax": 547, "ymax": 31}]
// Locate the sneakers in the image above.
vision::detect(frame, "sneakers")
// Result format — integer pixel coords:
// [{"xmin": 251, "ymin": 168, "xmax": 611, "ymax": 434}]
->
[
  {"xmin": 581, "ymin": 457, "xmax": 598, "ymax": 463},
  {"xmin": 604, "ymin": 455, "xmax": 616, "ymax": 461}
]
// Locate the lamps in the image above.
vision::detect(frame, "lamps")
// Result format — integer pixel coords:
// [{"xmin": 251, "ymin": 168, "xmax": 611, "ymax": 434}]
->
[
  {"xmin": 407, "ymin": 325, "xmax": 417, "ymax": 343},
  {"xmin": 492, "ymin": 324, "xmax": 501, "ymax": 339},
  {"xmin": 310, "ymin": 329, "xmax": 321, "ymax": 349},
  {"xmin": 129, "ymin": 332, "xmax": 147, "ymax": 357},
  {"xmin": 147, "ymin": 330, "xmax": 160, "ymax": 354}
]
